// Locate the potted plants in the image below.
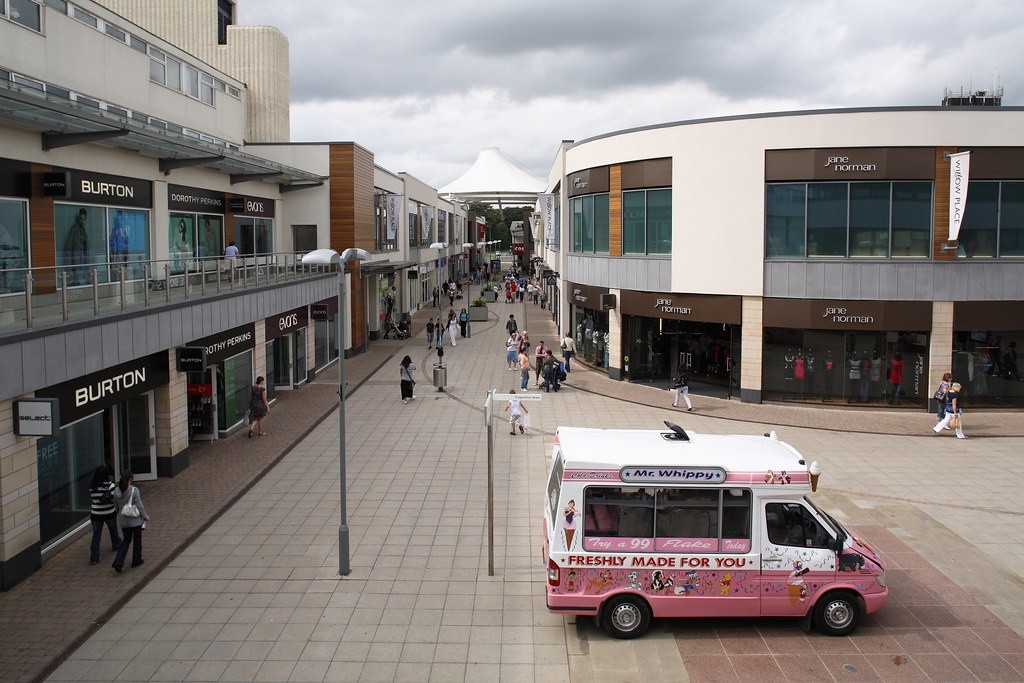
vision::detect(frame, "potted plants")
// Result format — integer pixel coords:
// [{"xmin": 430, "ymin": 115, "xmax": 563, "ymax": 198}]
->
[
  {"xmin": 484, "ymin": 285, "xmax": 495, "ymax": 303},
  {"xmin": 491, "ymin": 272, "xmax": 503, "ymax": 290}
]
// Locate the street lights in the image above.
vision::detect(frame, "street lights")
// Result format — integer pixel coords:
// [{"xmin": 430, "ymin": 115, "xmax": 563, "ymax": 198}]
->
[
  {"xmin": 301, "ymin": 248, "xmax": 372, "ymax": 574},
  {"xmin": 493, "ymin": 240, "xmax": 498, "ymax": 275},
  {"xmin": 487, "ymin": 241, "xmax": 493, "ymax": 285},
  {"xmin": 429, "ymin": 242, "xmax": 447, "ymax": 392},
  {"xmin": 462, "ymin": 243, "xmax": 475, "ymax": 338},
  {"xmin": 498, "ymin": 240, "xmax": 501, "ymax": 263}
]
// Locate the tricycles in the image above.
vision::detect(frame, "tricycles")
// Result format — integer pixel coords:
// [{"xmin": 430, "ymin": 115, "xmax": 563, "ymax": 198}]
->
[{"xmin": 505, "ymin": 297, "xmax": 513, "ymax": 303}]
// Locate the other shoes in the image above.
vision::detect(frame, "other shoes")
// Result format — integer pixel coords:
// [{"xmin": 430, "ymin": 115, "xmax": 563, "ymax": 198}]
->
[
  {"xmin": 258, "ymin": 433, "xmax": 266, "ymax": 436},
  {"xmin": 112, "ymin": 538, "xmax": 123, "ymax": 551},
  {"xmin": 944, "ymin": 425, "xmax": 951, "ymax": 429},
  {"xmin": 90, "ymin": 556, "xmax": 101, "ymax": 565},
  {"xmin": 932, "ymin": 428, "xmax": 939, "ymax": 436},
  {"xmin": 959, "ymin": 435, "xmax": 968, "ymax": 439},
  {"xmin": 514, "ymin": 367, "xmax": 517, "ymax": 371},
  {"xmin": 688, "ymin": 408, "xmax": 692, "ymax": 411},
  {"xmin": 521, "ymin": 387, "xmax": 527, "ymax": 392},
  {"xmin": 672, "ymin": 404, "xmax": 676, "ymax": 407},
  {"xmin": 519, "ymin": 425, "xmax": 524, "ymax": 434},
  {"xmin": 509, "ymin": 367, "xmax": 512, "ymax": 370},
  {"xmin": 131, "ymin": 559, "xmax": 144, "ymax": 568},
  {"xmin": 404, "ymin": 400, "xmax": 408, "ymax": 403},
  {"xmin": 410, "ymin": 396, "xmax": 416, "ymax": 400},
  {"xmin": 112, "ymin": 564, "xmax": 122, "ymax": 574},
  {"xmin": 248, "ymin": 428, "xmax": 253, "ymax": 438},
  {"xmin": 510, "ymin": 431, "xmax": 515, "ymax": 435}
]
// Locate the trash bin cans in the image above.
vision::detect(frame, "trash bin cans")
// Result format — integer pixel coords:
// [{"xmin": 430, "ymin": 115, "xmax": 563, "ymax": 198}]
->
[{"xmin": 435, "ymin": 368, "xmax": 447, "ymax": 387}]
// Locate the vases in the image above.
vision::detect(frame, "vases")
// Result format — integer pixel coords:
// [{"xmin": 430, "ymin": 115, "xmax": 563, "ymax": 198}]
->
[{"xmin": 469, "ymin": 304, "xmax": 488, "ymax": 322}]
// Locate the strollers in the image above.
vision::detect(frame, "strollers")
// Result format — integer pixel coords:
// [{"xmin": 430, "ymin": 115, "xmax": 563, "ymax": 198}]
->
[
  {"xmin": 392, "ymin": 323, "xmax": 408, "ymax": 340},
  {"xmin": 539, "ymin": 362, "xmax": 564, "ymax": 390}
]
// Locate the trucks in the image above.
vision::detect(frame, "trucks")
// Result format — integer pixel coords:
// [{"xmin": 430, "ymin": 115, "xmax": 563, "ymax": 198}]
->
[{"xmin": 542, "ymin": 421, "xmax": 889, "ymax": 641}]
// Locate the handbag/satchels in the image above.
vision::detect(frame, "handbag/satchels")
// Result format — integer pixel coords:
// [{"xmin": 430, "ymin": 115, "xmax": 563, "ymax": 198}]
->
[
  {"xmin": 243, "ymin": 408, "xmax": 251, "ymax": 427},
  {"xmin": 934, "ymin": 391, "xmax": 945, "ymax": 400},
  {"xmin": 559, "ymin": 362, "xmax": 565, "ymax": 372},
  {"xmin": 561, "ymin": 340, "xmax": 567, "ymax": 349},
  {"xmin": 99, "ymin": 481, "xmax": 114, "ymax": 504},
  {"xmin": 950, "ymin": 417, "xmax": 959, "ymax": 427},
  {"xmin": 121, "ymin": 487, "xmax": 140, "ymax": 517},
  {"xmin": 522, "ymin": 412, "xmax": 530, "ymax": 430},
  {"xmin": 675, "ymin": 380, "xmax": 684, "ymax": 387},
  {"xmin": 411, "ymin": 380, "xmax": 416, "ymax": 387}
]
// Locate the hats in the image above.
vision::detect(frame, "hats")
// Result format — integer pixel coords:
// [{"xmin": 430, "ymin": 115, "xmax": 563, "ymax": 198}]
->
[
  {"xmin": 523, "ymin": 330, "xmax": 528, "ymax": 336},
  {"xmin": 512, "ymin": 333, "xmax": 517, "ymax": 338}
]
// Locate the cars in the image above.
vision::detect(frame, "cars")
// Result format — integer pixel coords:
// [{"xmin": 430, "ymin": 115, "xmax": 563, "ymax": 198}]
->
[
  {"xmin": 496, "ymin": 251, "xmax": 501, "ymax": 256},
  {"xmin": 952, "ymin": 351, "xmax": 995, "ymax": 373}
]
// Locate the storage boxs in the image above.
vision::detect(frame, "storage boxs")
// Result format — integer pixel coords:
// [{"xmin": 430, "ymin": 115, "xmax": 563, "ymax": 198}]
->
[{"xmin": 851, "ymin": 231, "xmax": 929, "ymax": 256}]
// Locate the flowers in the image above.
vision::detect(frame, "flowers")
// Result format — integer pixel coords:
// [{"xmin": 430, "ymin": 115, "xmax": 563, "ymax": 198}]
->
[{"xmin": 472, "ymin": 295, "xmax": 487, "ymax": 307}]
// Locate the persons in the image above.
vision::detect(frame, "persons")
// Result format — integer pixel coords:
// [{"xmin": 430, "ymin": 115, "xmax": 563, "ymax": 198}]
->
[
  {"xmin": 172, "ymin": 218, "xmax": 188, "ymax": 272},
  {"xmin": 578, "ymin": 314, "xmax": 609, "ymax": 370},
  {"xmin": 504, "ymin": 390, "xmax": 529, "ymax": 435},
  {"xmin": 89, "ymin": 466, "xmax": 123, "ymax": 565},
  {"xmin": 66, "ymin": 208, "xmax": 93, "ymax": 287},
  {"xmin": 247, "ymin": 376, "xmax": 270, "ymax": 438},
  {"xmin": 953, "ymin": 330, "xmax": 1024, "ymax": 381},
  {"xmin": 400, "ymin": 355, "xmax": 416, "ymax": 404},
  {"xmin": 205, "ymin": 219, "xmax": 217, "ymax": 259},
  {"xmin": 112, "ymin": 470, "xmax": 149, "ymax": 574},
  {"xmin": 646, "ymin": 329, "xmax": 729, "ymax": 379},
  {"xmin": 383, "ymin": 258, "xmax": 576, "ymax": 393},
  {"xmin": 672, "ymin": 363, "xmax": 692, "ymax": 411},
  {"xmin": 784, "ymin": 350, "xmax": 904, "ymax": 405},
  {"xmin": 223, "ymin": 240, "xmax": 238, "ymax": 283},
  {"xmin": 108, "ymin": 211, "xmax": 129, "ymax": 282},
  {"xmin": 931, "ymin": 372, "xmax": 968, "ymax": 438}
]
